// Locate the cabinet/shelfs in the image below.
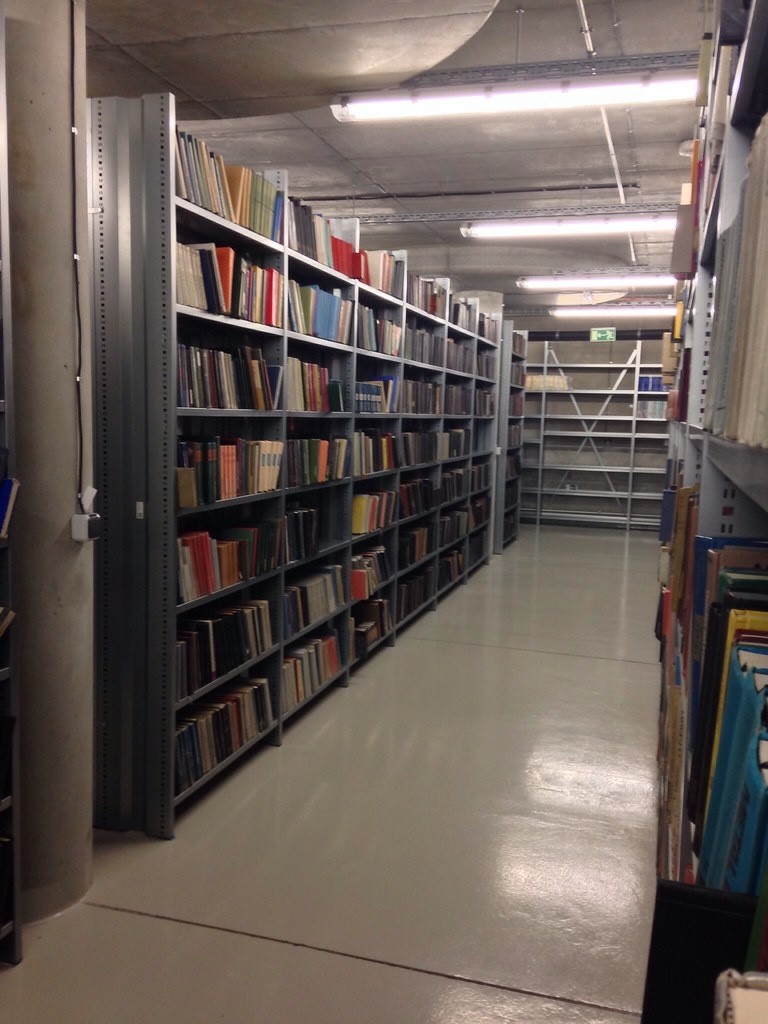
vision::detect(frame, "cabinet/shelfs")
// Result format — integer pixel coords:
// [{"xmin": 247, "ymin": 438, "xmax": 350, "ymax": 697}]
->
[{"xmin": 87, "ymin": 0, "xmax": 768, "ymax": 881}]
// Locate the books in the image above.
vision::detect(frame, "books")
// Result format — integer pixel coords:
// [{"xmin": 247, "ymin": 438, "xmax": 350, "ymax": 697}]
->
[
  {"xmin": 0, "ymin": 124, "xmax": 570, "ymax": 792},
  {"xmin": 634, "ymin": 114, "xmax": 768, "ymax": 897}
]
